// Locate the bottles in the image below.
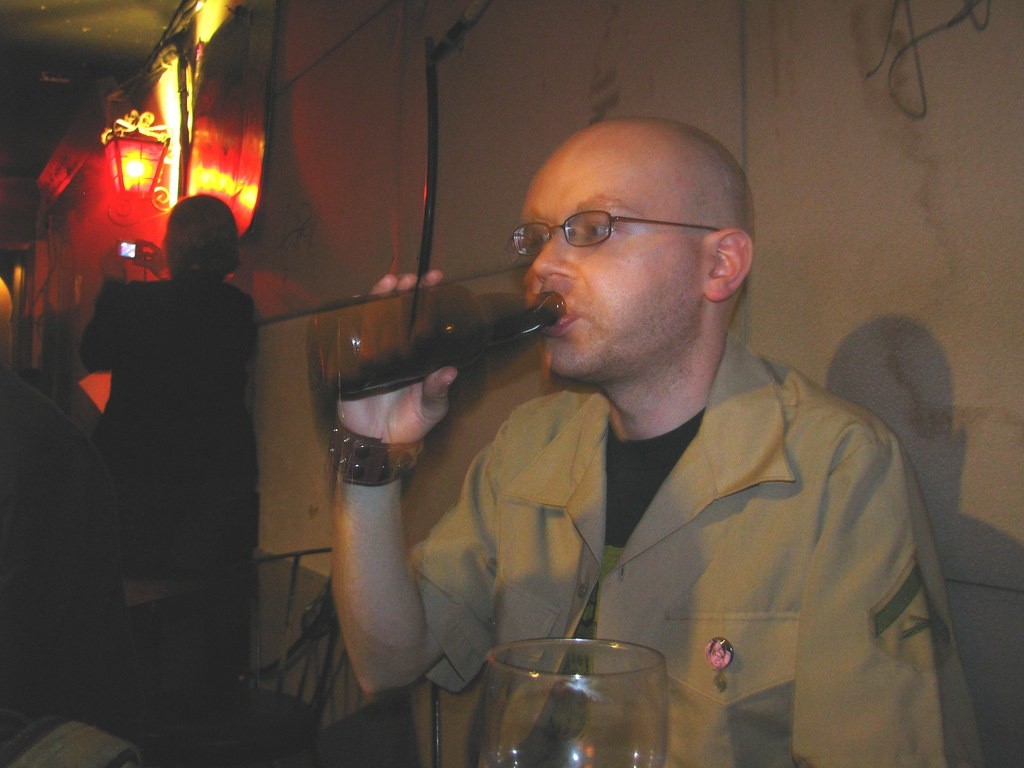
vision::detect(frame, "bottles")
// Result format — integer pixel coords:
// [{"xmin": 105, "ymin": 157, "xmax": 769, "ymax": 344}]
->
[{"xmin": 307, "ymin": 284, "xmax": 565, "ymax": 395}]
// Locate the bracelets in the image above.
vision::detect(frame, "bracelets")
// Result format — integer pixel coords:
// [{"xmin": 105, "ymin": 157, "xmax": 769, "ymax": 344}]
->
[{"xmin": 330, "ymin": 417, "xmax": 424, "ymax": 486}]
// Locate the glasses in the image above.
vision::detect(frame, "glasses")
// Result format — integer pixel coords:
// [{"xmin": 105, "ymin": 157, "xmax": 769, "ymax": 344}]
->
[{"xmin": 511, "ymin": 210, "xmax": 721, "ymax": 256}]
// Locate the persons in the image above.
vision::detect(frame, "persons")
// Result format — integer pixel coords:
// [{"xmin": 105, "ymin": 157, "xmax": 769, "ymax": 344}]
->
[
  {"xmin": 0, "ymin": 195, "xmax": 257, "ymax": 737},
  {"xmin": 329, "ymin": 115, "xmax": 982, "ymax": 768}
]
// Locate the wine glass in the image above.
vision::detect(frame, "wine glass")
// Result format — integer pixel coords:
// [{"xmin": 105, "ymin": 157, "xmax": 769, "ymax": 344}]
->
[{"xmin": 466, "ymin": 637, "xmax": 669, "ymax": 768}]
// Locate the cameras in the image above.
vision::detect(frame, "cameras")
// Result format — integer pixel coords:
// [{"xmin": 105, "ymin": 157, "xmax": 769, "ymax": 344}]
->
[{"xmin": 116, "ymin": 241, "xmax": 141, "ymax": 261}]
